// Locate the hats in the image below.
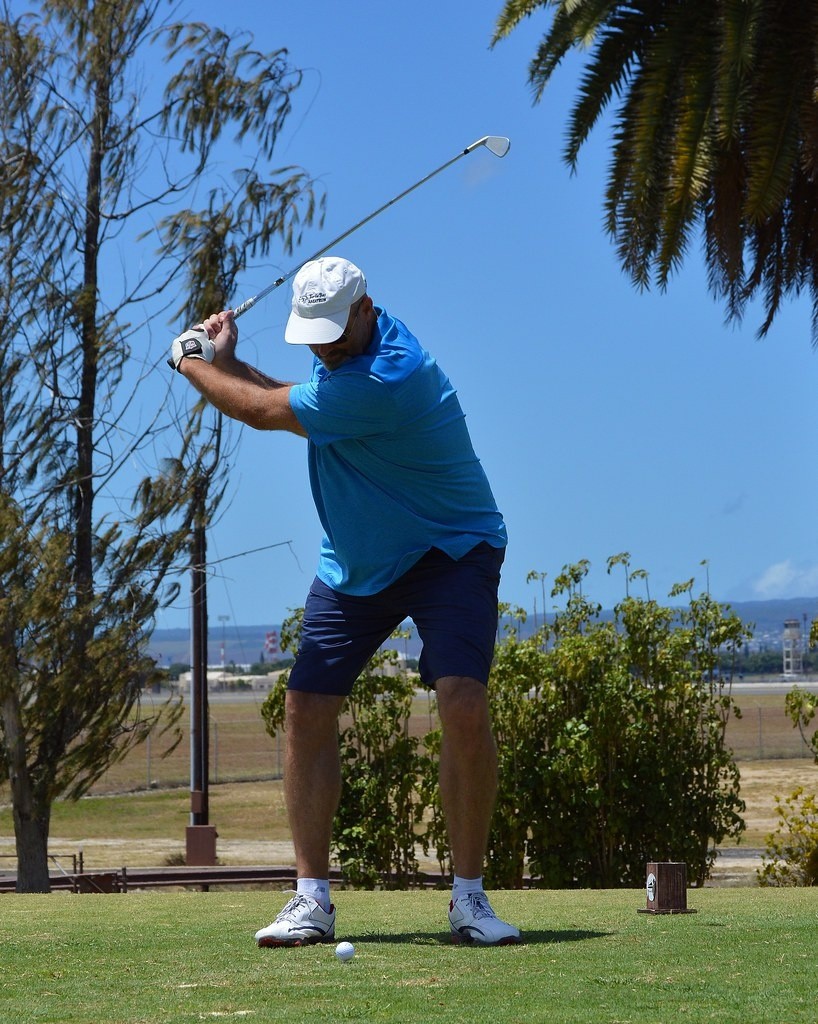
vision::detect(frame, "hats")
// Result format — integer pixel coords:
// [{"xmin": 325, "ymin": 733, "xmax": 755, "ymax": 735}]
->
[{"xmin": 284, "ymin": 257, "xmax": 366, "ymax": 346}]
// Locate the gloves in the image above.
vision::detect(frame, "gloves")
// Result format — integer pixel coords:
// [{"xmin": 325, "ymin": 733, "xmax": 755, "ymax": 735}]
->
[{"xmin": 171, "ymin": 324, "xmax": 216, "ymax": 374}]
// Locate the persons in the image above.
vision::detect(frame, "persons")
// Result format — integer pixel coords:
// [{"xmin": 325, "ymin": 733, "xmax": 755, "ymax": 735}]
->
[{"xmin": 170, "ymin": 257, "xmax": 525, "ymax": 946}]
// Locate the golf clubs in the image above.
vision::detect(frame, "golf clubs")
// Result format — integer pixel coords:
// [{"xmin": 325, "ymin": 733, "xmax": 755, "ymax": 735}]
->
[{"xmin": 166, "ymin": 134, "xmax": 510, "ymax": 368}]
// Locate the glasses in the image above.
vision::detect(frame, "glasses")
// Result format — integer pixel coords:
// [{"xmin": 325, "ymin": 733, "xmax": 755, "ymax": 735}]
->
[{"xmin": 331, "ymin": 300, "xmax": 364, "ymax": 347}]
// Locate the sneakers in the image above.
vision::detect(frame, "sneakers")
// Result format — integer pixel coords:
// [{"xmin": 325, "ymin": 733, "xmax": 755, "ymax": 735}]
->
[
  {"xmin": 254, "ymin": 887, "xmax": 337, "ymax": 946},
  {"xmin": 448, "ymin": 892, "xmax": 519, "ymax": 944}
]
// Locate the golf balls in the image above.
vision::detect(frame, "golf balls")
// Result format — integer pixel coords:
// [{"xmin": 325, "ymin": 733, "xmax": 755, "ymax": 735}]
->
[{"xmin": 335, "ymin": 942, "xmax": 354, "ymax": 961}]
